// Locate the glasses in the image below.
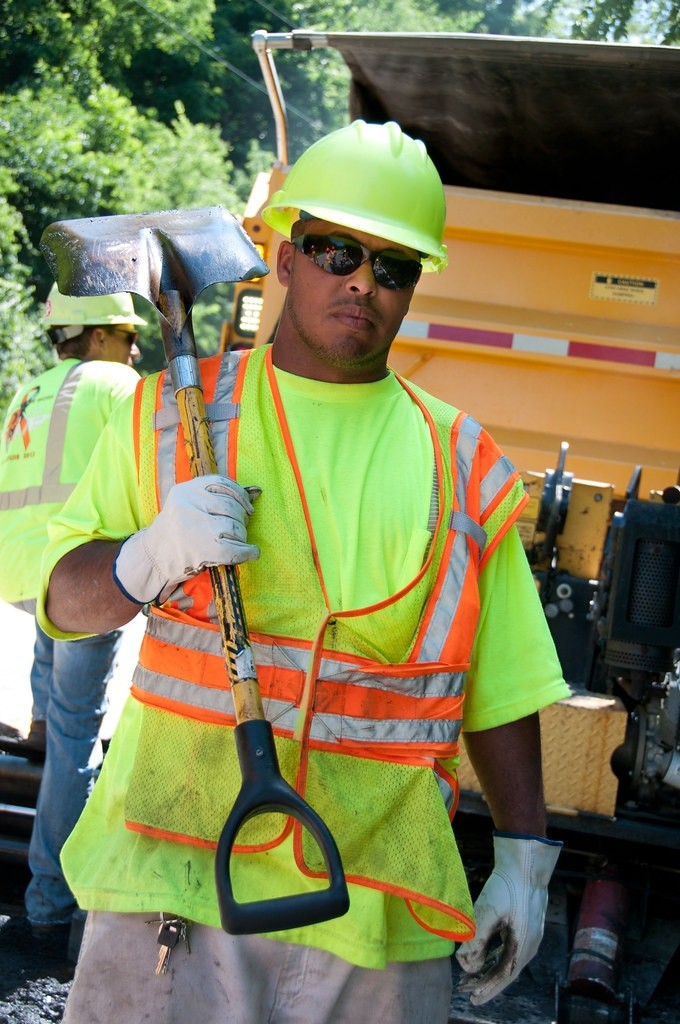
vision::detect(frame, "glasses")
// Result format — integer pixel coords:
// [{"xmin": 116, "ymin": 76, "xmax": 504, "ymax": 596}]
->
[
  {"xmin": 104, "ymin": 327, "xmax": 138, "ymax": 347},
  {"xmin": 291, "ymin": 234, "xmax": 423, "ymax": 291}
]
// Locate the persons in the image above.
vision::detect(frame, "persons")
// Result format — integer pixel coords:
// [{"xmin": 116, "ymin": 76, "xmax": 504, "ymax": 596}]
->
[
  {"xmin": 0, "ymin": 278, "xmax": 150, "ymax": 941},
  {"xmin": 34, "ymin": 117, "xmax": 576, "ymax": 1024}
]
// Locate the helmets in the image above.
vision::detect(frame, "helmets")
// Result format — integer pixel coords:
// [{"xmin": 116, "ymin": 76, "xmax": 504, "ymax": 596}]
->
[
  {"xmin": 38, "ymin": 280, "xmax": 148, "ymax": 327},
  {"xmin": 261, "ymin": 119, "xmax": 449, "ymax": 275}
]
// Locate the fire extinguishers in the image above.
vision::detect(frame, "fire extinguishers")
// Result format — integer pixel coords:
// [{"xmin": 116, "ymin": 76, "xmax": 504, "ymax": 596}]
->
[{"xmin": 564, "ymin": 878, "xmax": 630, "ymax": 1004}]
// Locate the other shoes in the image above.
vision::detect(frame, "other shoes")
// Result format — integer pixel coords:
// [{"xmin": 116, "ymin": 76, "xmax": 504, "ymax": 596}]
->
[
  {"xmin": 32, "ymin": 922, "xmax": 68, "ymax": 942},
  {"xmin": 26, "ymin": 721, "xmax": 46, "ymax": 758}
]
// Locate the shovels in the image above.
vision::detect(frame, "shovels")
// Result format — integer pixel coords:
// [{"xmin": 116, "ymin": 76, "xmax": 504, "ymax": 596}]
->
[{"xmin": 40, "ymin": 203, "xmax": 352, "ymax": 938}]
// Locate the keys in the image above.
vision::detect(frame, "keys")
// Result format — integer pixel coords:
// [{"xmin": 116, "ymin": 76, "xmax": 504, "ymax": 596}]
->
[{"xmin": 143, "ymin": 910, "xmax": 193, "ymax": 976}]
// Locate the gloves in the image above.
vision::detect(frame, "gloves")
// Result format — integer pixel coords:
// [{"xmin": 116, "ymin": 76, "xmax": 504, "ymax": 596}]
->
[
  {"xmin": 457, "ymin": 828, "xmax": 564, "ymax": 1005},
  {"xmin": 113, "ymin": 473, "xmax": 263, "ymax": 607}
]
